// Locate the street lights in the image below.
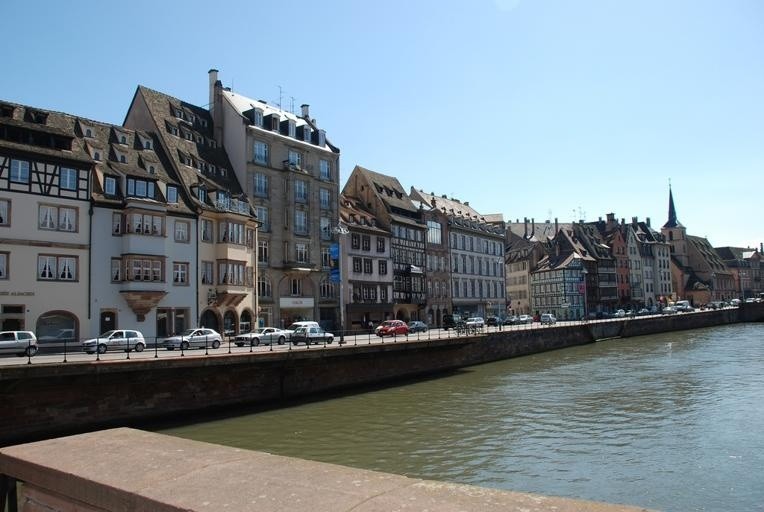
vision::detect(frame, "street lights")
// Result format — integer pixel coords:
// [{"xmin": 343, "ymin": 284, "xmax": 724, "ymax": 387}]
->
[{"xmin": 335, "ymin": 225, "xmax": 349, "ymax": 344}]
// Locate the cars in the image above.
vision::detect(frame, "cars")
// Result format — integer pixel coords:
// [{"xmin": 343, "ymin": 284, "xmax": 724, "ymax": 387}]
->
[
  {"xmin": 0, "ymin": 331, "xmax": 38, "ymax": 356},
  {"xmin": 41, "ymin": 329, "xmax": 75, "ymax": 342},
  {"xmin": 230, "ymin": 322, "xmax": 250, "ymax": 335},
  {"xmin": 442, "ymin": 314, "xmax": 533, "ymax": 330},
  {"xmin": 375, "ymin": 320, "xmax": 428, "ymax": 337},
  {"xmin": 540, "ymin": 314, "xmax": 556, "ymax": 324},
  {"xmin": 83, "ymin": 330, "xmax": 146, "ymax": 354},
  {"xmin": 588, "ymin": 297, "xmax": 694, "ymax": 319},
  {"xmin": 707, "ymin": 298, "xmax": 763, "ymax": 309},
  {"xmin": 235, "ymin": 322, "xmax": 334, "ymax": 346},
  {"xmin": 163, "ymin": 328, "xmax": 222, "ymax": 350}
]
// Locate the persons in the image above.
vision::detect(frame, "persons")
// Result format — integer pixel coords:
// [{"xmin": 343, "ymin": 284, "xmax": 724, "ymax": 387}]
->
[{"xmin": 368, "ymin": 320, "xmax": 373, "ymax": 334}]
